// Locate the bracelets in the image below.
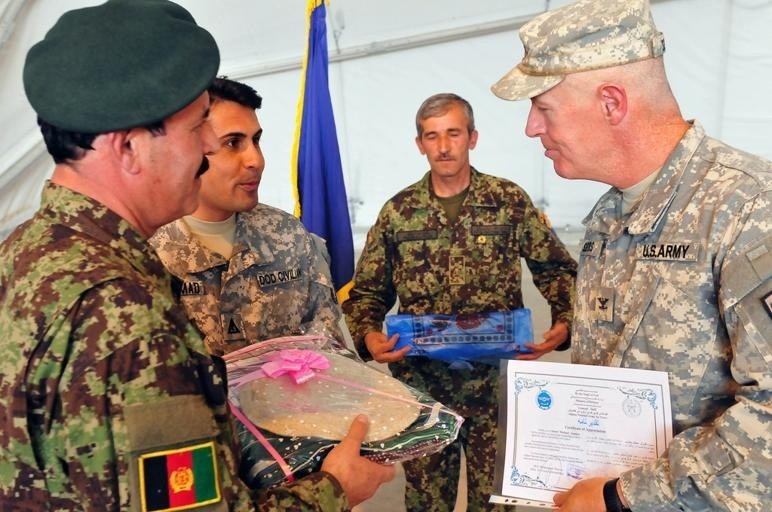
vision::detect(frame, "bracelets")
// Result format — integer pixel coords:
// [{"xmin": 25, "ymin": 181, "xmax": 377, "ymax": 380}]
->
[{"xmin": 601, "ymin": 479, "xmax": 630, "ymax": 512}]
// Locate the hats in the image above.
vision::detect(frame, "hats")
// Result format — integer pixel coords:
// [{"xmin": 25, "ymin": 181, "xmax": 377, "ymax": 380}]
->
[
  {"xmin": 491, "ymin": 0, "xmax": 665, "ymax": 101},
  {"xmin": 23, "ymin": 0, "xmax": 221, "ymax": 131}
]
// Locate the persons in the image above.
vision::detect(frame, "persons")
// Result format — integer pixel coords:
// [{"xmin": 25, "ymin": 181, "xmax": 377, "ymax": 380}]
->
[
  {"xmin": 0, "ymin": 0, "xmax": 395, "ymax": 512},
  {"xmin": 339, "ymin": 93, "xmax": 580, "ymax": 512},
  {"xmin": 493, "ymin": 0, "xmax": 772, "ymax": 512},
  {"xmin": 146, "ymin": 78, "xmax": 346, "ymax": 490}
]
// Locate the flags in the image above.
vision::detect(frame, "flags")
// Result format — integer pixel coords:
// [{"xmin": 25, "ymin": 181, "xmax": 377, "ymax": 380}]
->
[{"xmin": 293, "ymin": 0, "xmax": 356, "ymax": 305}]
